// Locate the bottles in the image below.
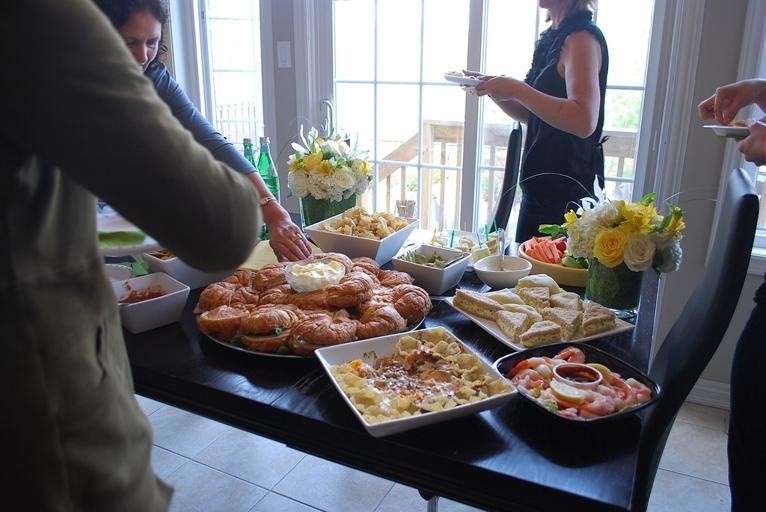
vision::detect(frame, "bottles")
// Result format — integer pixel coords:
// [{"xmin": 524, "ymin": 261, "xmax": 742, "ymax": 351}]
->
[
  {"xmin": 241, "ymin": 138, "xmax": 257, "ymax": 168},
  {"xmin": 257, "ymin": 137, "xmax": 282, "ymax": 240}
]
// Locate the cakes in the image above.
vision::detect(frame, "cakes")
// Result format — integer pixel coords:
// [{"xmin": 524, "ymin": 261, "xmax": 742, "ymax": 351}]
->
[{"xmin": 429, "ymin": 231, "xmax": 500, "ymax": 262}]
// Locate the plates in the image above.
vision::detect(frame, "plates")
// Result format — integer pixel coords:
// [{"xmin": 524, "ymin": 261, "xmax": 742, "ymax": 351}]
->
[
  {"xmin": 314, "ymin": 326, "xmax": 518, "ymax": 440},
  {"xmin": 703, "ymin": 124, "xmax": 753, "ymax": 139},
  {"xmin": 444, "ymin": 295, "xmax": 637, "ymax": 350},
  {"xmin": 519, "ymin": 235, "xmax": 589, "ymax": 290},
  {"xmin": 444, "ymin": 73, "xmax": 484, "ymax": 87},
  {"xmin": 497, "ymin": 342, "xmax": 665, "ymax": 426},
  {"xmin": 422, "ymin": 230, "xmax": 513, "ymax": 275}
]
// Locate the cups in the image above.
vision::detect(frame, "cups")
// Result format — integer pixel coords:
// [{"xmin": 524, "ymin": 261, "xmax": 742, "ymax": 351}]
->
[{"xmin": 551, "ymin": 363, "xmax": 603, "ymax": 404}]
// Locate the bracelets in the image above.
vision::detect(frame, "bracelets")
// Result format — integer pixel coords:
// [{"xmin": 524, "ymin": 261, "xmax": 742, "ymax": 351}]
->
[{"xmin": 259, "ymin": 195, "xmax": 278, "ymax": 205}]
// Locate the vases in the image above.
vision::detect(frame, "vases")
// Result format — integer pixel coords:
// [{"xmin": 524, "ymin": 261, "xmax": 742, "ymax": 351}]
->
[
  {"xmin": 300, "ymin": 191, "xmax": 357, "ymax": 225},
  {"xmin": 583, "ymin": 255, "xmax": 642, "ymax": 319}
]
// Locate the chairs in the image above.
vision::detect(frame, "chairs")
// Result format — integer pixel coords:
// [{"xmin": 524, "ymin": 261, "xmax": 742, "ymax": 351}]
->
[{"xmin": 636, "ymin": 167, "xmax": 760, "ymax": 512}]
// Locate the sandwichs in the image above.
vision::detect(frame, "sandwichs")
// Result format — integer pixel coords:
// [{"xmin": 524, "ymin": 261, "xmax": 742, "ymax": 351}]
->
[{"xmin": 453, "ymin": 274, "xmax": 617, "ymax": 348}]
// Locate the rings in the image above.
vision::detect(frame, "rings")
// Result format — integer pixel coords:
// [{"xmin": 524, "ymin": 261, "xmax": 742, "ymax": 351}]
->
[{"xmin": 292, "ymin": 232, "xmax": 303, "ymax": 242}]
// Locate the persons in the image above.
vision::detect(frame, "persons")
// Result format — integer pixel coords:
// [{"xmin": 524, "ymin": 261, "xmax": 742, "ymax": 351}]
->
[
  {"xmin": 695, "ymin": 78, "xmax": 765, "ymax": 512},
  {"xmin": 94, "ymin": 0, "xmax": 312, "ymax": 267},
  {"xmin": 460, "ymin": 0, "xmax": 612, "ymax": 252},
  {"xmin": 2, "ymin": 0, "xmax": 261, "ymax": 511}
]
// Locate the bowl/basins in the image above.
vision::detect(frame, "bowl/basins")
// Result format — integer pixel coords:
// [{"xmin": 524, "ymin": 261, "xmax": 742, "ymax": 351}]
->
[
  {"xmin": 475, "ymin": 254, "xmax": 531, "ymax": 288},
  {"xmin": 111, "ymin": 271, "xmax": 190, "ymax": 334},
  {"xmin": 285, "ymin": 259, "xmax": 345, "ymax": 294},
  {"xmin": 392, "ymin": 243, "xmax": 473, "ymax": 293},
  {"xmin": 305, "ymin": 209, "xmax": 418, "ymax": 269},
  {"xmin": 144, "ymin": 251, "xmax": 206, "ymax": 292}
]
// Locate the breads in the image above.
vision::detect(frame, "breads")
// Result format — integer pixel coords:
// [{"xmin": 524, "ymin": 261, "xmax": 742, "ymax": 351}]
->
[{"xmin": 197, "ymin": 252, "xmax": 433, "ymax": 356}]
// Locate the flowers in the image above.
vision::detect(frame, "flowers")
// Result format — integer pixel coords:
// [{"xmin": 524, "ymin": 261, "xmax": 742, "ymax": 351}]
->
[
  {"xmin": 539, "ymin": 173, "xmax": 687, "ymax": 274},
  {"xmin": 286, "ymin": 125, "xmax": 371, "ymax": 201}
]
